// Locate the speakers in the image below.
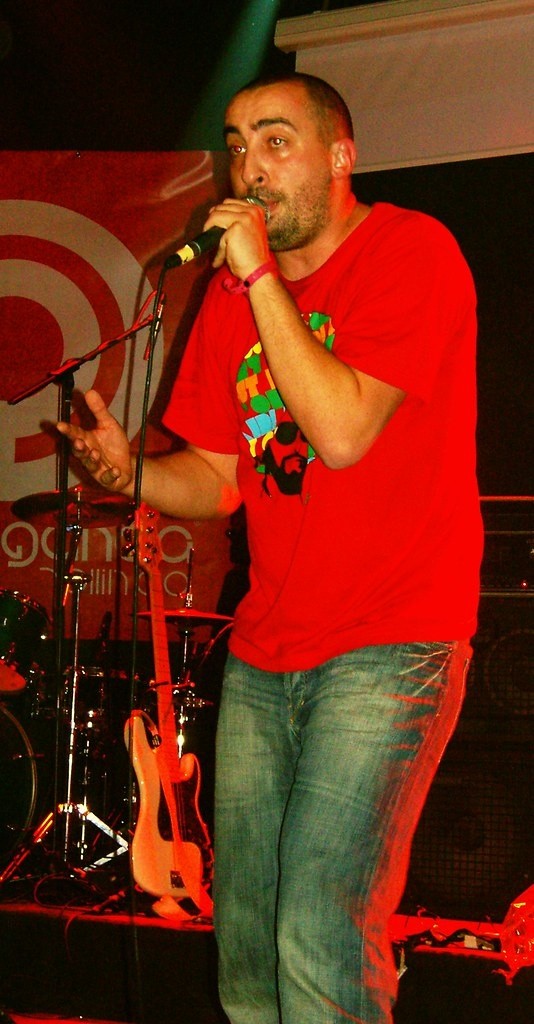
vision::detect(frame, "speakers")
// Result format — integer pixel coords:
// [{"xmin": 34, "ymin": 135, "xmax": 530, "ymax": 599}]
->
[{"xmin": 389, "ymin": 494, "xmax": 534, "ymax": 923}]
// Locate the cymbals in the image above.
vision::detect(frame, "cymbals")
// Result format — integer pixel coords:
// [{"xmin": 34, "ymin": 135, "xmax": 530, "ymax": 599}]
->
[
  {"xmin": 11, "ymin": 489, "xmax": 136, "ymax": 530},
  {"xmin": 130, "ymin": 607, "xmax": 235, "ymax": 623}
]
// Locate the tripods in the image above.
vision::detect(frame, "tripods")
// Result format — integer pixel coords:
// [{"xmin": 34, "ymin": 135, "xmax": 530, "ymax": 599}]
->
[{"xmin": 0, "ymin": 514, "xmax": 138, "ymax": 910}]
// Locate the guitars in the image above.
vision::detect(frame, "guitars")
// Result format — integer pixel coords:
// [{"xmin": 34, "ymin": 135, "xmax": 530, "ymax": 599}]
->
[
  {"xmin": 84, "ymin": 609, "xmax": 116, "ymax": 764},
  {"xmin": 120, "ymin": 498, "xmax": 215, "ymax": 900}
]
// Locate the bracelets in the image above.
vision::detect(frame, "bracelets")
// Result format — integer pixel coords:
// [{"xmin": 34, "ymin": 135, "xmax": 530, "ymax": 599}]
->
[{"xmin": 222, "ymin": 261, "xmax": 275, "ymax": 295}]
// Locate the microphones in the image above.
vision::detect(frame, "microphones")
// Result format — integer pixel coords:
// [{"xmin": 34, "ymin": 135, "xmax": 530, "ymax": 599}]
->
[
  {"xmin": 166, "ymin": 195, "xmax": 272, "ymax": 270},
  {"xmin": 96, "ymin": 611, "xmax": 112, "ymax": 645}
]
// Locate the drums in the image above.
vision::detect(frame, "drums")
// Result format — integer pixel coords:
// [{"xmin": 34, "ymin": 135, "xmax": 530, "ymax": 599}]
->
[
  {"xmin": 0, "ymin": 587, "xmax": 51, "ymax": 694},
  {"xmin": 0, "ymin": 695, "xmax": 134, "ymax": 862},
  {"xmin": 61, "ymin": 663, "xmax": 149, "ymax": 732}
]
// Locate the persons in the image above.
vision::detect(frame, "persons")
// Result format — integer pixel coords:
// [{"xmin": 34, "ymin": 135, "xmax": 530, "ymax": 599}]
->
[{"xmin": 57, "ymin": 71, "xmax": 484, "ymax": 1024}]
